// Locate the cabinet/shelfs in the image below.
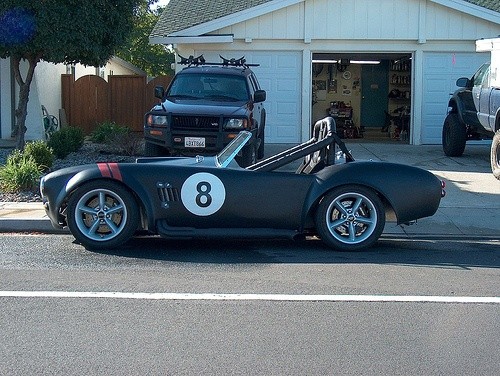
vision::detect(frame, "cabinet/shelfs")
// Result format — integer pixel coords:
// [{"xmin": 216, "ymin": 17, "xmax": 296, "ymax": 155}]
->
[{"xmin": 389, "ymin": 58, "xmax": 411, "ymax": 117}]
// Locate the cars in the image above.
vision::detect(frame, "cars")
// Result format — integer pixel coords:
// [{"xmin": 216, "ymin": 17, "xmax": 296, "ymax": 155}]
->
[{"xmin": 37, "ymin": 119, "xmax": 444, "ymax": 254}]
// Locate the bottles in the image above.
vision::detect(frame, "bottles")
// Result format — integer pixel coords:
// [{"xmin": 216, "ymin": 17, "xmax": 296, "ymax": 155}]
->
[
  {"xmin": 404, "ymin": 130, "xmax": 408, "ymax": 141},
  {"xmin": 399, "ymin": 129, "xmax": 404, "ymax": 140}
]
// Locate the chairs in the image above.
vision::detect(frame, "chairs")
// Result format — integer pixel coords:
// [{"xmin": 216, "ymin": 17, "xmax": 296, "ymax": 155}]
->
[
  {"xmin": 295, "ymin": 152, "xmax": 314, "ymax": 175},
  {"xmin": 302, "ymin": 147, "xmax": 325, "ymax": 175}
]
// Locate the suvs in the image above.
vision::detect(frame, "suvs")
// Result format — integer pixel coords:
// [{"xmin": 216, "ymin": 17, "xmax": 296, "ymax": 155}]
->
[
  {"xmin": 441, "ymin": 62, "xmax": 500, "ymax": 179},
  {"xmin": 143, "ymin": 53, "xmax": 267, "ymax": 168}
]
